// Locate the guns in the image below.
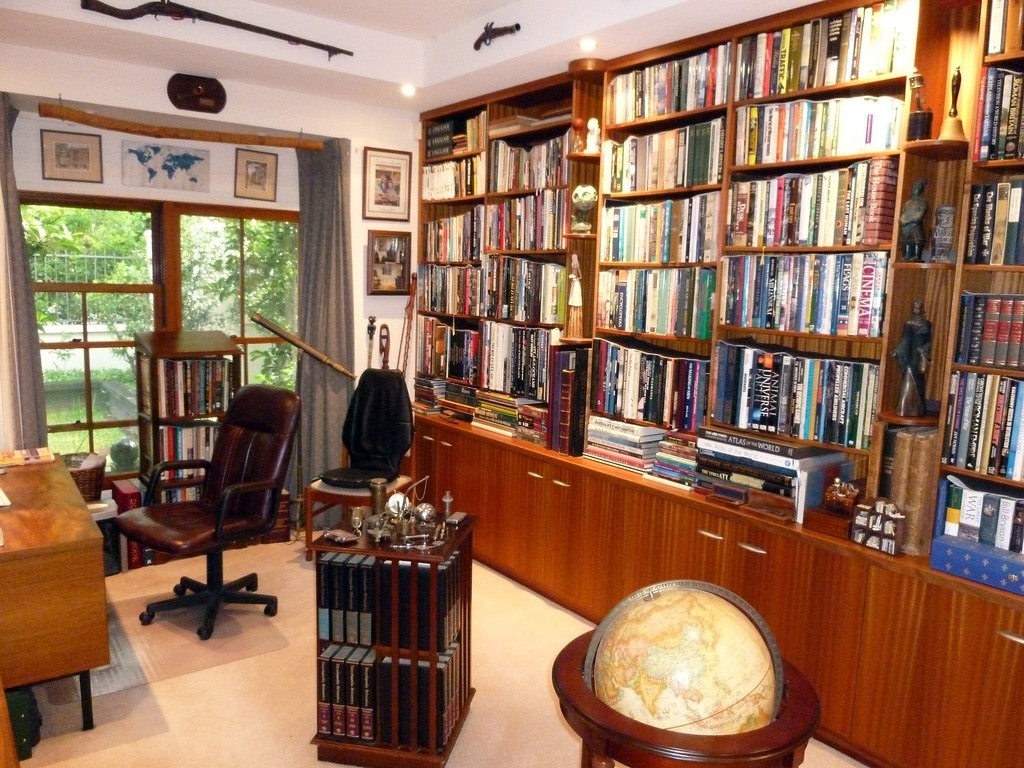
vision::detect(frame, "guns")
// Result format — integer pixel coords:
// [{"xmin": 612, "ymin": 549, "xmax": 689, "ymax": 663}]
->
[
  {"xmin": 473, "ymin": 21, "xmax": 521, "ymax": 51},
  {"xmin": 81, "ymin": 0, "xmax": 356, "ymax": 57}
]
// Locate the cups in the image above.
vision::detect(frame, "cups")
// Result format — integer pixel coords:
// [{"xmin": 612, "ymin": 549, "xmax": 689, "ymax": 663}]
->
[{"xmin": 371, "ymin": 478, "xmax": 387, "ymax": 513}]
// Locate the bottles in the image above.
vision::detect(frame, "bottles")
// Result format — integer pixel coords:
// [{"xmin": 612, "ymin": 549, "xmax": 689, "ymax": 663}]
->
[{"xmin": 443, "ymin": 491, "xmax": 453, "ymax": 520}]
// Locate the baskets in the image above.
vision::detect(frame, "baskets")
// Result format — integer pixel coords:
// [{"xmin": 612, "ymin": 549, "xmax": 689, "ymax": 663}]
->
[{"xmin": 62, "ymin": 452, "xmax": 106, "ymax": 502}]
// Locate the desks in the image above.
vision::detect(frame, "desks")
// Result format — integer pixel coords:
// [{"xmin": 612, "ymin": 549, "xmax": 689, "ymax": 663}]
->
[{"xmin": 0, "ymin": 452, "xmax": 110, "ymax": 731}]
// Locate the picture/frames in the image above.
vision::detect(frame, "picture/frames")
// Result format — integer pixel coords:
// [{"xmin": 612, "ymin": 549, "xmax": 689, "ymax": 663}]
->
[
  {"xmin": 367, "ymin": 229, "xmax": 412, "ymax": 296},
  {"xmin": 234, "ymin": 146, "xmax": 279, "ymax": 203},
  {"xmin": 40, "ymin": 128, "xmax": 104, "ymax": 184},
  {"xmin": 362, "ymin": 146, "xmax": 413, "ymax": 223}
]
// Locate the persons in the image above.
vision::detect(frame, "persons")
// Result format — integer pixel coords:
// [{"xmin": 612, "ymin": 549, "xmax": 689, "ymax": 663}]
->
[
  {"xmin": 372, "ymin": 237, "xmax": 406, "ymax": 292},
  {"xmin": 380, "ymin": 173, "xmax": 397, "ymax": 205},
  {"xmin": 889, "ymin": 298, "xmax": 930, "ymax": 419},
  {"xmin": 899, "ymin": 178, "xmax": 928, "ymax": 260}
]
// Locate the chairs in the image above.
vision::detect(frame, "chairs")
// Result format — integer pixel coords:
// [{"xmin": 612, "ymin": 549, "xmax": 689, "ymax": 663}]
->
[
  {"xmin": 304, "ymin": 442, "xmax": 413, "ymax": 560},
  {"xmin": 114, "ymin": 383, "xmax": 301, "ymax": 640}
]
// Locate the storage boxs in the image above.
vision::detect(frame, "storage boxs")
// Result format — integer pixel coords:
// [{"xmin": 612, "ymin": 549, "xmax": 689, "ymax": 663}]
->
[
  {"xmin": 706, "ymin": 483, "xmax": 796, "ymax": 527},
  {"xmin": 928, "ymin": 535, "xmax": 1024, "ymax": 597},
  {"xmin": 801, "ymin": 508, "xmax": 852, "ymax": 541}
]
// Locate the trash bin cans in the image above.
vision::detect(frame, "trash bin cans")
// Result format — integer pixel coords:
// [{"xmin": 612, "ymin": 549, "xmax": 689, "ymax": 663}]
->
[
  {"xmin": 86, "ymin": 499, "xmax": 121, "ymax": 576},
  {"xmin": 61, "ymin": 447, "xmax": 110, "ymax": 502}
]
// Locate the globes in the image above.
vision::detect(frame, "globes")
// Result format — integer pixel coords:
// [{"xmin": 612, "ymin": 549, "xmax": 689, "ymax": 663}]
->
[{"xmin": 552, "ymin": 580, "xmax": 821, "ymax": 768}]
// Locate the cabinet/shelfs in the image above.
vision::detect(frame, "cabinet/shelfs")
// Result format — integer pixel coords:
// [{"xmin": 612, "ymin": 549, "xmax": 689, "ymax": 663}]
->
[
  {"xmin": 135, "ymin": 330, "xmax": 246, "ymax": 565},
  {"xmin": 396, "ymin": 1, "xmax": 1023, "ymax": 768},
  {"xmin": 310, "ymin": 515, "xmax": 478, "ymax": 768}
]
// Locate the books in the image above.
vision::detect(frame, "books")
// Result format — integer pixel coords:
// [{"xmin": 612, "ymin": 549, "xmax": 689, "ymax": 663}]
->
[
  {"xmin": 157, "ymin": 358, "xmax": 234, "ymax": 502},
  {"xmin": 0, "ymin": 445, "xmax": 55, "ymax": 468},
  {"xmin": 317, "ymin": 554, "xmax": 467, "ymax": 754},
  {"xmin": 112, "ymin": 477, "xmax": 155, "ymax": 570},
  {"xmin": 414, "ymin": 1, "xmax": 1024, "ymax": 553}
]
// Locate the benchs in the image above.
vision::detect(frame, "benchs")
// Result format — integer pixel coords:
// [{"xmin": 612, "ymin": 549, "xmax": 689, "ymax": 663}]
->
[{"xmin": 45, "ymin": 378, "xmax": 138, "ymax": 420}]
